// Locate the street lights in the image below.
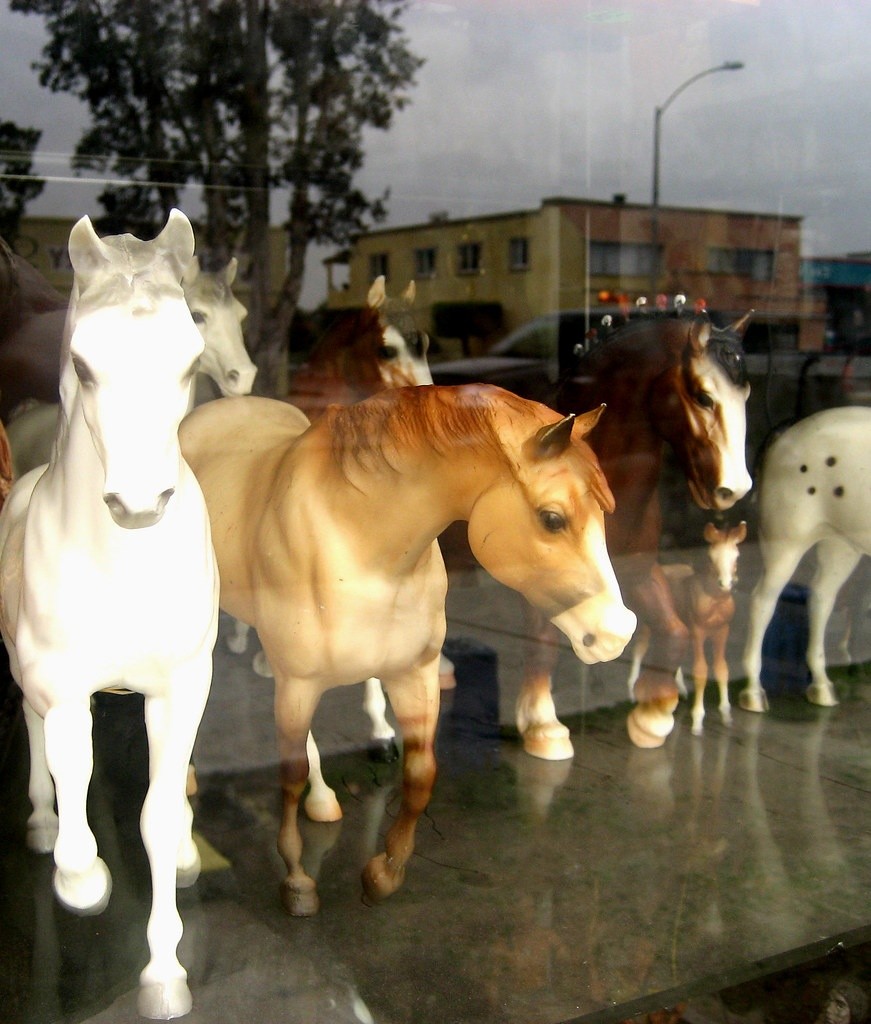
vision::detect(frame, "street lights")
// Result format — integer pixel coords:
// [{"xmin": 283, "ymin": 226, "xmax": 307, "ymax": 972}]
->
[{"xmin": 650, "ymin": 62, "xmax": 751, "ymax": 298}]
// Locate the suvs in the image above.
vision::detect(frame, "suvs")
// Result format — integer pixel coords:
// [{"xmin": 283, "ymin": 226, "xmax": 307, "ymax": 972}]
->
[{"xmin": 429, "ymin": 304, "xmax": 725, "ymax": 419}]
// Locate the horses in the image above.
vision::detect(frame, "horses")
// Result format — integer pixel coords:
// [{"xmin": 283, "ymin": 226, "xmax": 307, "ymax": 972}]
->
[{"xmin": 1, "ymin": 208, "xmax": 870, "ymax": 1022}]
